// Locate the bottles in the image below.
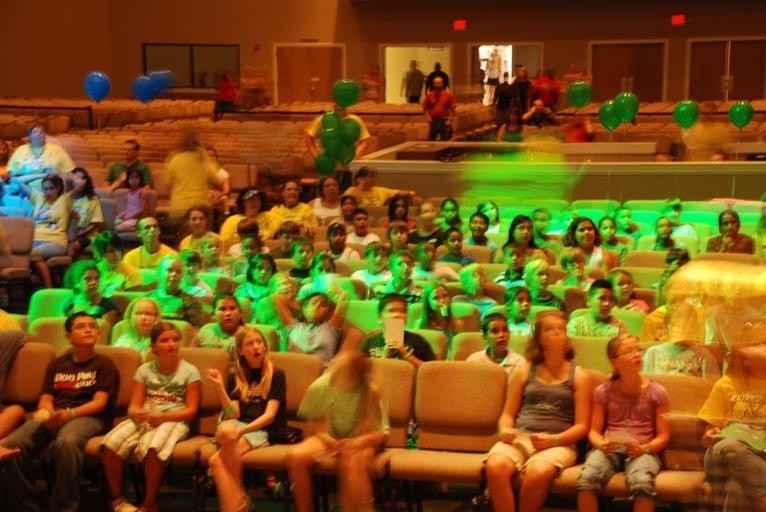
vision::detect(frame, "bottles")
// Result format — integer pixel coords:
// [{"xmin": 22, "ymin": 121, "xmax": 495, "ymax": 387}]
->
[{"xmin": 406, "ymin": 417, "xmax": 417, "ymax": 447}]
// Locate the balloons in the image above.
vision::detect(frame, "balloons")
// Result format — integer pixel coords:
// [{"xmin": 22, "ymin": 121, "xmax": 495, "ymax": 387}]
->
[
  {"xmin": 672, "ymin": 99, "xmax": 698, "ymax": 128},
  {"xmin": 134, "ymin": 70, "xmax": 172, "ymax": 102},
  {"xmin": 314, "ymin": 81, "xmax": 358, "ymax": 176},
  {"xmin": 599, "ymin": 91, "xmax": 639, "ymax": 131},
  {"xmin": 729, "ymin": 100, "xmax": 754, "ymax": 127},
  {"xmin": 85, "ymin": 70, "xmax": 110, "ymax": 103}
]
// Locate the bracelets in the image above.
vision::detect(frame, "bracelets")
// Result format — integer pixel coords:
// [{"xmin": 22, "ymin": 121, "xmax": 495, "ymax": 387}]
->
[
  {"xmin": 552, "ymin": 434, "xmax": 560, "ymax": 446},
  {"xmin": 67, "ymin": 408, "xmax": 75, "ymax": 420}
]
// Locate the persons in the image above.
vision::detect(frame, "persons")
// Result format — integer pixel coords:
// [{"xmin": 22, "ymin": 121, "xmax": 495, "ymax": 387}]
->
[
  {"xmin": 207, "ymin": 327, "xmax": 288, "ymax": 511},
  {"xmin": 0, "ymin": 311, "xmax": 120, "ymax": 511},
  {"xmin": 98, "ymin": 323, "xmax": 203, "ymax": 511},
  {"xmin": 213, "ymin": 70, "xmax": 236, "ymax": 114},
  {"xmin": 303, "ymin": 101, "xmax": 370, "ymax": 192},
  {"xmin": 698, "ymin": 318, "xmax": 766, "ymax": 511},
  {"xmin": 68, "ymin": 160, "xmax": 766, "ymax": 383},
  {"xmin": 0, "ymin": 329, "xmax": 30, "ymax": 461},
  {"xmin": 287, "ymin": 349, "xmax": 391, "ymax": 512},
  {"xmin": 400, "ymin": 59, "xmax": 454, "ymax": 142},
  {"xmin": 482, "ymin": 310, "xmax": 594, "ymax": 511},
  {"xmin": 1, "ymin": 122, "xmax": 230, "ymax": 287},
  {"xmin": 479, "ymin": 45, "xmax": 596, "ymax": 142},
  {"xmin": 576, "ymin": 333, "xmax": 674, "ymax": 511}
]
